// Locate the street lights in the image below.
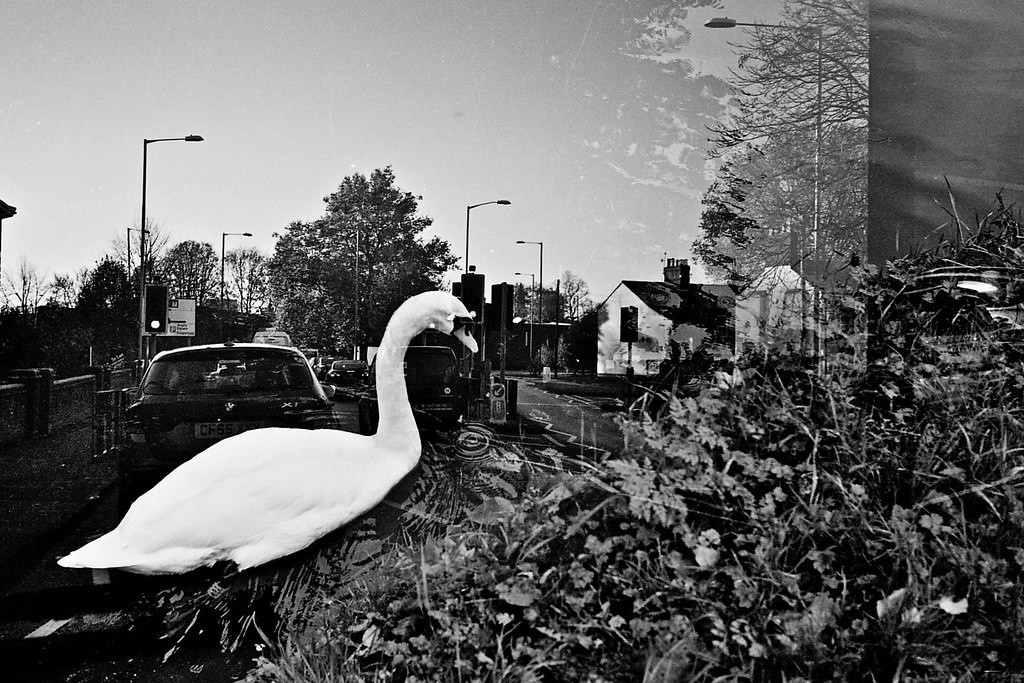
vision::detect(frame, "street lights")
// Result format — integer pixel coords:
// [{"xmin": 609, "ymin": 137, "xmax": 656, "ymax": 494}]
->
[
  {"xmin": 704, "ymin": 14, "xmax": 825, "ymax": 369},
  {"xmin": 515, "ymin": 272, "xmax": 534, "ymax": 366},
  {"xmin": 516, "ymin": 241, "xmax": 543, "ymax": 375},
  {"xmin": 466, "ymin": 198, "xmax": 512, "ymax": 272},
  {"xmin": 137, "ymin": 133, "xmax": 204, "ymax": 380},
  {"xmin": 126, "ymin": 227, "xmax": 150, "ymax": 282},
  {"xmin": 532, "ymin": 278, "xmax": 560, "ymax": 378},
  {"xmin": 220, "ymin": 233, "xmax": 254, "ymax": 309}
]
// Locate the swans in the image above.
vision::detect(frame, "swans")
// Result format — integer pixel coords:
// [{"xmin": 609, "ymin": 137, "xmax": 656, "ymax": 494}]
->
[{"xmin": 57, "ymin": 290, "xmax": 479, "ymax": 575}]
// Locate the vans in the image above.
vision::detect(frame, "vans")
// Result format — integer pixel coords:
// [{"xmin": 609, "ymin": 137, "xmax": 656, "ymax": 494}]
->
[{"xmin": 250, "ymin": 327, "xmax": 294, "ymax": 347}]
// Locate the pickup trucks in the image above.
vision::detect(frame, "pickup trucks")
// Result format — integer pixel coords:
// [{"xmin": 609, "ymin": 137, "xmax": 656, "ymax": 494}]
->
[{"xmin": 355, "ymin": 345, "xmax": 518, "ymax": 438}]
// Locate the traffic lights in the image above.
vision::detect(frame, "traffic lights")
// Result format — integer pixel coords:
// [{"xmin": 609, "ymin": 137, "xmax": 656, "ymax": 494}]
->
[
  {"xmin": 452, "ymin": 282, "xmax": 463, "ymax": 296},
  {"xmin": 141, "ymin": 283, "xmax": 168, "ymax": 337},
  {"xmin": 620, "ymin": 306, "xmax": 640, "ymax": 344},
  {"xmin": 459, "ymin": 273, "xmax": 484, "ymax": 325}
]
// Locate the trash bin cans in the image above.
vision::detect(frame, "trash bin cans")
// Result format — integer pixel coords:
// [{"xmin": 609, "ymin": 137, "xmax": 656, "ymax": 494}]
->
[
  {"xmin": 493, "ymin": 375, "xmax": 519, "ymax": 417},
  {"xmin": 543, "ymin": 367, "xmax": 551, "ymax": 382}
]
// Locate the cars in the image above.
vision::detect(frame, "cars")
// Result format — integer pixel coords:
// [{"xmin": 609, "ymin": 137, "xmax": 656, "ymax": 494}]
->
[
  {"xmin": 6, "ymin": 366, "xmax": 57, "ymax": 385},
  {"xmin": 122, "ymin": 342, "xmax": 340, "ymax": 477},
  {"xmin": 307, "ymin": 356, "xmax": 344, "ymax": 381},
  {"xmin": 325, "ymin": 360, "xmax": 370, "ymax": 383}
]
